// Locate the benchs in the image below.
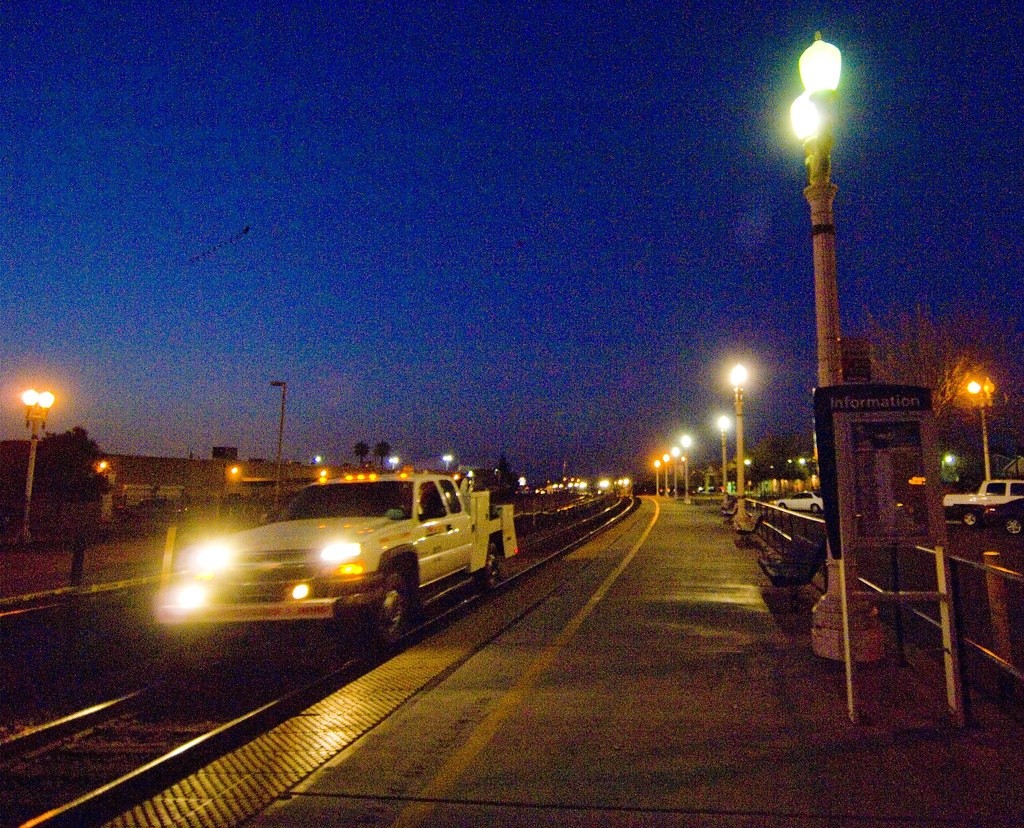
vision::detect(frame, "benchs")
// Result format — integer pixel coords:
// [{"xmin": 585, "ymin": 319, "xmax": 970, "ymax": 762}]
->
[
  {"xmin": 758, "ymin": 536, "xmax": 822, "ymax": 606},
  {"xmin": 720, "ymin": 505, "xmax": 739, "ymax": 525},
  {"xmin": 732, "ymin": 513, "xmax": 763, "ymax": 548}
]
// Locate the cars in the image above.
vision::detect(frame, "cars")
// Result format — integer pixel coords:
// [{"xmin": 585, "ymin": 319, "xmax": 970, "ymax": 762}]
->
[
  {"xmin": 987, "ymin": 498, "xmax": 1024, "ymax": 536},
  {"xmin": 769, "ymin": 490, "xmax": 826, "ymax": 514}
]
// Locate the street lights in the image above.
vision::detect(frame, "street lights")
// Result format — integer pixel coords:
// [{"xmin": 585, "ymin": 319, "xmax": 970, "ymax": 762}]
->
[
  {"xmin": 270, "ymin": 379, "xmax": 288, "ymax": 512},
  {"xmin": 654, "ymin": 460, "xmax": 662, "ymax": 497},
  {"xmin": 728, "ymin": 363, "xmax": 755, "ymax": 531},
  {"xmin": 12, "ymin": 388, "xmax": 57, "ymax": 545},
  {"xmin": 672, "ymin": 447, "xmax": 680, "ymax": 502},
  {"xmin": 718, "ymin": 415, "xmax": 731, "ymax": 493},
  {"xmin": 681, "ymin": 437, "xmax": 693, "ymax": 504},
  {"xmin": 788, "ymin": 27, "xmax": 886, "ymax": 668},
  {"xmin": 661, "ymin": 453, "xmax": 670, "ymax": 499},
  {"xmin": 966, "ymin": 376, "xmax": 994, "ymax": 484}
]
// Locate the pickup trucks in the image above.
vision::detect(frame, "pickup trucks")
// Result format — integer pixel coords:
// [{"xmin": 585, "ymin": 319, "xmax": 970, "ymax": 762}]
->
[
  {"xmin": 940, "ymin": 477, "xmax": 1024, "ymax": 530},
  {"xmin": 155, "ymin": 472, "xmax": 522, "ymax": 647}
]
